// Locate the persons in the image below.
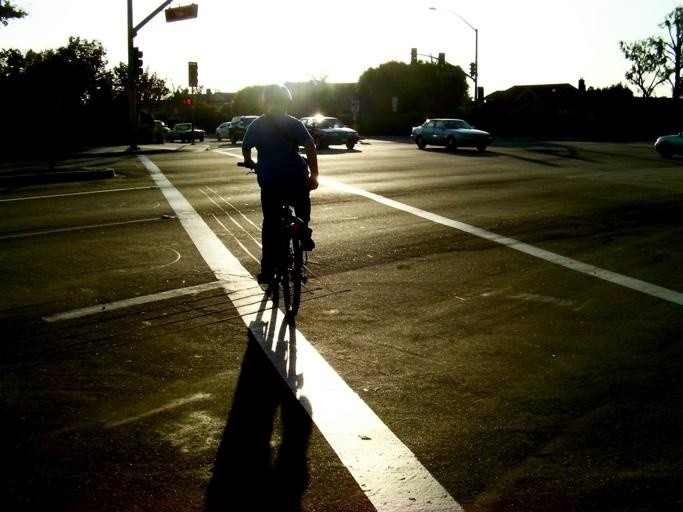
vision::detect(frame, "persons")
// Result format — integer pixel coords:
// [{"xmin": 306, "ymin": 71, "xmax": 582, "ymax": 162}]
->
[{"xmin": 239, "ymin": 81, "xmax": 319, "ymax": 286}]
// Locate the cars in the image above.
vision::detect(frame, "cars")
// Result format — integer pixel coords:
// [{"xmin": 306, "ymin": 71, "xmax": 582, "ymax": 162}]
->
[
  {"xmin": 653, "ymin": 130, "xmax": 682, "ymax": 160},
  {"xmin": 151, "ymin": 114, "xmax": 360, "ymax": 152},
  {"xmin": 408, "ymin": 116, "xmax": 493, "ymax": 154}
]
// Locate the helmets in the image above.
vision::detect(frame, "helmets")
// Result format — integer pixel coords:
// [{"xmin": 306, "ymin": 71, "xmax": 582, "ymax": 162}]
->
[{"xmin": 262, "ymin": 83, "xmax": 293, "ymax": 104}]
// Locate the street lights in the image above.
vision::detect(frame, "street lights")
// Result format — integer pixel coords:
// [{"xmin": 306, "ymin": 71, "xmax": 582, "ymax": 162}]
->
[{"xmin": 429, "ymin": 5, "xmax": 479, "ymax": 102}]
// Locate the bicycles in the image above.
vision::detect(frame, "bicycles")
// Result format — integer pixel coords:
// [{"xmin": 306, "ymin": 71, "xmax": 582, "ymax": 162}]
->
[{"xmin": 228, "ymin": 157, "xmax": 308, "ymax": 318}]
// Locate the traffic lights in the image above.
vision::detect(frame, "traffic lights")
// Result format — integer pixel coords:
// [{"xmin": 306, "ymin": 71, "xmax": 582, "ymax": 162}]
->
[
  {"xmin": 131, "ymin": 46, "xmax": 144, "ymax": 78},
  {"xmin": 411, "ymin": 49, "xmax": 417, "ymax": 66},
  {"xmin": 438, "ymin": 52, "xmax": 446, "ymax": 70},
  {"xmin": 188, "ymin": 60, "xmax": 199, "ymax": 88},
  {"xmin": 468, "ymin": 61, "xmax": 476, "ymax": 77}
]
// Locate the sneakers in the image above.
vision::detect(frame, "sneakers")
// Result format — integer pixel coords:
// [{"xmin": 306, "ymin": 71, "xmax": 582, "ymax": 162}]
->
[
  {"xmin": 257, "ymin": 267, "xmax": 278, "ymax": 285},
  {"xmin": 299, "ymin": 227, "xmax": 315, "ymax": 248}
]
